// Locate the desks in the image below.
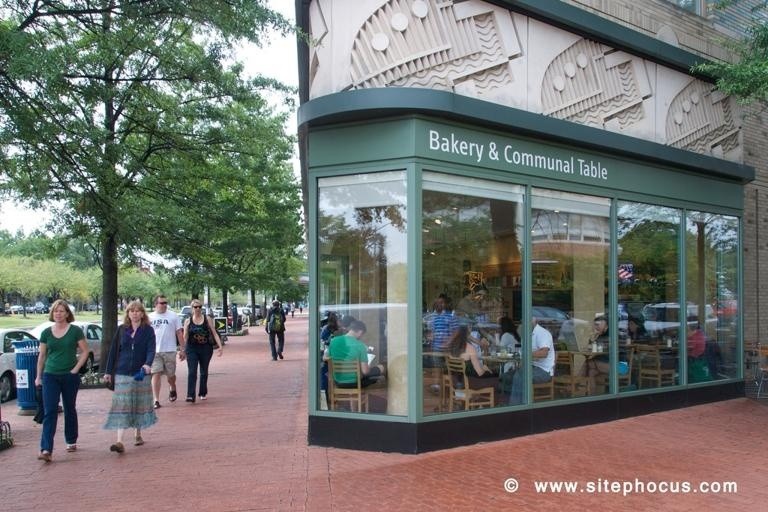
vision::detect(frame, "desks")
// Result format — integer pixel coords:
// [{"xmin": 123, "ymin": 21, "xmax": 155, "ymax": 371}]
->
[
  {"xmin": 554, "ymin": 348, "xmax": 609, "ymax": 376},
  {"xmin": 421, "ymin": 352, "xmax": 522, "ymax": 398}
]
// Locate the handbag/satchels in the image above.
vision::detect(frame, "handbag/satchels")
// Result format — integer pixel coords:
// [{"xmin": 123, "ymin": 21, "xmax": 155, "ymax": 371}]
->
[
  {"xmin": 106, "ymin": 323, "xmax": 123, "ymax": 391},
  {"xmin": 37, "ymin": 452, "xmax": 53, "ymax": 462}
]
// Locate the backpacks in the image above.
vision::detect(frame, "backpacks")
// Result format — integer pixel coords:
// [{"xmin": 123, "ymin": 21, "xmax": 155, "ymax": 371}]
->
[{"xmin": 270, "ymin": 307, "xmax": 283, "ymax": 333}]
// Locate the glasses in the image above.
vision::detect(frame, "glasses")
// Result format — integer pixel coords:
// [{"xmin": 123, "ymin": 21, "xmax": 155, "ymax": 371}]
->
[
  {"xmin": 159, "ymin": 301, "xmax": 168, "ymax": 305},
  {"xmin": 193, "ymin": 305, "xmax": 202, "ymax": 309}
]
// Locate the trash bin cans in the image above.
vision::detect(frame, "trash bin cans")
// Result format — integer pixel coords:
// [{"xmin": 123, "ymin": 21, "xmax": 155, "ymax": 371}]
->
[{"xmin": 10, "ymin": 339, "xmax": 62, "ymax": 417}]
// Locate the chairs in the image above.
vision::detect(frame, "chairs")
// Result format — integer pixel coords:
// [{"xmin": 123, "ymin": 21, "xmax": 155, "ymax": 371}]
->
[
  {"xmin": 328, "ymin": 357, "xmax": 369, "ymax": 413},
  {"xmin": 533, "ymin": 348, "xmax": 557, "ymax": 402},
  {"xmin": 636, "ymin": 345, "xmax": 675, "ymax": 386},
  {"xmin": 597, "ymin": 346, "xmax": 635, "ymax": 391},
  {"xmin": 444, "ymin": 355, "xmax": 494, "ymax": 413},
  {"xmin": 557, "ymin": 350, "xmax": 592, "ymax": 399}
]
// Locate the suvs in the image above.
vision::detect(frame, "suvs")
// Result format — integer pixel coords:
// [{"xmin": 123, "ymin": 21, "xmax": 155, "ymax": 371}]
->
[
  {"xmin": 617, "ymin": 302, "xmax": 718, "ymax": 343},
  {"xmin": 246, "ymin": 304, "xmax": 261, "ymax": 317},
  {"xmin": 179, "ymin": 306, "xmax": 213, "ymax": 319},
  {"xmin": 712, "ymin": 299, "xmax": 738, "ymax": 325},
  {"xmin": 214, "ymin": 307, "xmax": 223, "ymax": 316}
]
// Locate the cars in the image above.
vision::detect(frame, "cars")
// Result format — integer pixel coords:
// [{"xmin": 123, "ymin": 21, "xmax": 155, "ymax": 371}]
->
[
  {"xmin": 25, "ymin": 321, "xmax": 103, "ymax": 372},
  {"xmin": 532, "ymin": 306, "xmax": 571, "ymax": 336},
  {"xmin": 421, "ymin": 313, "xmax": 500, "ymax": 347},
  {"xmin": 1, "ymin": 328, "xmax": 40, "ymax": 404}
]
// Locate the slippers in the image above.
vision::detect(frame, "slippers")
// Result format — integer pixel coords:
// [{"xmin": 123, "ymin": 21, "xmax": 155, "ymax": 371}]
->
[
  {"xmin": 154, "ymin": 400, "xmax": 160, "ymax": 409},
  {"xmin": 168, "ymin": 384, "xmax": 177, "ymax": 402}
]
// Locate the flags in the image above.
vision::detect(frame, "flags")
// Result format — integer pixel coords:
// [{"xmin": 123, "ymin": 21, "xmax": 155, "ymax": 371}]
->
[{"xmin": 617, "ymin": 266, "xmax": 632, "ymax": 280}]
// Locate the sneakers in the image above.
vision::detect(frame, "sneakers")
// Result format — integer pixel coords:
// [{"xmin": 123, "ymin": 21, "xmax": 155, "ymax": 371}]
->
[
  {"xmin": 272, "ymin": 357, "xmax": 278, "ymax": 360},
  {"xmin": 110, "ymin": 441, "xmax": 125, "ymax": 452},
  {"xmin": 134, "ymin": 436, "xmax": 144, "ymax": 445},
  {"xmin": 277, "ymin": 351, "xmax": 284, "ymax": 359},
  {"xmin": 66, "ymin": 445, "xmax": 76, "ymax": 452}
]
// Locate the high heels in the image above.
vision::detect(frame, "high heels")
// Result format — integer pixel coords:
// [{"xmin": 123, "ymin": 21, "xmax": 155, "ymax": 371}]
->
[
  {"xmin": 200, "ymin": 392, "xmax": 208, "ymax": 401},
  {"xmin": 186, "ymin": 394, "xmax": 196, "ymax": 403}
]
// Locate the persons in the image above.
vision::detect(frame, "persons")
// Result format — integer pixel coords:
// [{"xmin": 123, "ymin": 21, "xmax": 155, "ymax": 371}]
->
[
  {"xmin": 291, "ymin": 300, "xmax": 295, "ymax": 318},
  {"xmin": 335, "ymin": 314, "xmax": 356, "ymax": 336},
  {"xmin": 457, "ymin": 280, "xmax": 489, "ymax": 320},
  {"xmin": 628, "ymin": 311, "xmax": 652, "ymax": 360},
  {"xmin": 667, "ymin": 315, "xmax": 706, "ymax": 380},
  {"xmin": 559, "ymin": 310, "xmax": 591, "ymax": 378},
  {"xmin": 320, "ymin": 311, "xmax": 342, "ymax": 390},
  {"xmin": 299, "ymin": 302, "xmax": 302, "ymax": 313},
  {"xmin": 510, "ymin": 317, "xmax": 555, "ymax": 406},
  {"xmin": 444, "ymin": 317, "xmax": 500, "ymax": 404},
  {"xmin": 148, "ymin": 295, "xmax": 187, "ymax": 409},
  {"xmin": 105, "ymin": 302, "xmax": 156, "ymax": 452},
  {"xmin": 324, "ymin": 321, "xmax": 388, "ymax": 388},
  {"xmin": 265, "ymin": 299, "xmax": 286, "ymax": 361},
  {"xmin": 427, "ymin": 293, "xmax": 456, "ymax": 394},
  {"xmin": 587, "ymin": 316, "xmax": 609, "ymax": 351},
  {"xmin": 179, "ymin": 298, "xmax": 223, "ymax": 403},
  {"xmin": 559, "ymin": 317, "xmax": 630, "ymax": 398},
  {"xmin": 496, "ymin": 316, "xmax": 521, "ymax": 397},
  {"xmin": 35, "ymin": 301, "xmax": 89, "ymax": 463}
]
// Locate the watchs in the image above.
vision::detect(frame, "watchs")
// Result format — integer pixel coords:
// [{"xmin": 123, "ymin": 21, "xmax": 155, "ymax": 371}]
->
[{"xmin": 182, "ymin": 349, "xmax": 186, "ymax": 352}]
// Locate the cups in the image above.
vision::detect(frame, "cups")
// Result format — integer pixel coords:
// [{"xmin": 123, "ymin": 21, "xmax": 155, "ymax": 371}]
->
[
  {"xmin": 506, "ymin": 353, "xmax": 514, "ymax": 357},
  {"xmin": 495, "ymin": 352, "xmax": 502, "ymax": 357}
]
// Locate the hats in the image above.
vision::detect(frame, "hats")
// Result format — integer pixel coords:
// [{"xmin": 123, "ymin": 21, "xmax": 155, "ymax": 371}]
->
[
  {"xmin": 627, "ymin": 311, "xmax": 645, "ymax": 324},
  {"xmin": 459, "ymin": 317, "xmax": 477, "ymax": 325}
]
